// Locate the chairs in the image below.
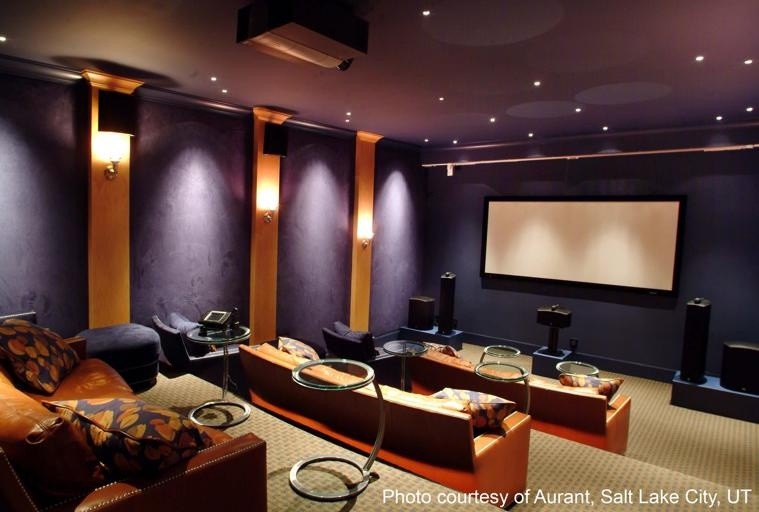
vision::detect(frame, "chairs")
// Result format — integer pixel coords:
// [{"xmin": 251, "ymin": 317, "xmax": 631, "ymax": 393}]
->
[
  {"xmin": 320, "ymin": 321, "xmax": 412, "ymax": 393},
  {"xmin": 152, "ymin": 311, "xmax": 241, "ymax": 372}
]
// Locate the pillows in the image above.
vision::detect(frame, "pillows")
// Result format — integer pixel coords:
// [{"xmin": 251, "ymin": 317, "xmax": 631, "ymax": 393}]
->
[
  {"xmin": 332, "ymin": 320, "xmax": 380, "ymax": 355},
  {"xmin": 423, "ymin": 341, "xmax": 462, "ymax": 360},
  {"xmin": 429, "ymin": 388, "xmax": 516, "ymax": 436},
  {"xmin": 167, "ymin": 312, "xmax": 210, "ymax": 357},
  {"xmin": 1, "ymin": 318, "xmax": 81, "ymax": 396},
  {"xmin": 40, "ymin": 398, "xmax": 215, "ymax": 480},
  {"xmin": 558, "ymin": 372, "xmax": 624, "ymax": 408},
  {"xmin": 277, "ymin": 335, "xmax": 323, "ymax": 363}
]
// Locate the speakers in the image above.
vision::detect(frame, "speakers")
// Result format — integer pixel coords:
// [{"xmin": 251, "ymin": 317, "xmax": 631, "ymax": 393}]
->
[
  {"xmin": 263, "ymin": 123, "xmax": 289, "ymax": 156},
  {"xmin": 536, "ymin": 304, "xmax": 572, "ymax": 328},
  {"xmin": 98, "ymin": 89, "xmax": 137, "ymax": 136},
  {"xmin": 407, "ymin": 296, "xmax": 435, "ymax": 330},
  {"xmin": 680, "ymin": 299, "xmax": 712, "ymax": 384},
  {"xmin": 720, "ymin": 341, "xmax": 759, "ymax": 395},
  {"xmin": 438, "ymin": 271, "xmax": 456, "ymax": 334}
]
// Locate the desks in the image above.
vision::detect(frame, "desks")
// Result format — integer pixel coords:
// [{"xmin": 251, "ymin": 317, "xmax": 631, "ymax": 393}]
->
[
  {"xmin": 288, "ymin": 359, "xmax": 386, "ymax": 501},
  {"xmin": 479, "ymin": 344, "xmax": 521, "ymax": 363},
  {"xmin": 187, "ymin": 325, "xmax": 251, "ymax": 428},
  {"xmin": 383, "ymin": 340, "xmax": 428, "ymax": 391},
  {"xmin": 556, "ymin": 361, "xmax": 599, "ymax": 379}
]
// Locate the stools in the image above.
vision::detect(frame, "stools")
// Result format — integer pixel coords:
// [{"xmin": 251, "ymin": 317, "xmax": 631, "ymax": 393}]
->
[{"xmin": 74, "ymin": 323, "xmax": 162, "ymax": 386}]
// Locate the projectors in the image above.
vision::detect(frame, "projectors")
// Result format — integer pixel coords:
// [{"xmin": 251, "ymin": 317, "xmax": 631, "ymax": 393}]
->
[{"xmin": 236, "ymin": 0, "xmax": 370, "ymax": 71}]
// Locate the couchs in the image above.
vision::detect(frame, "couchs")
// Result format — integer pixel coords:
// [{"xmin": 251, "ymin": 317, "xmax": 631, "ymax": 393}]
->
[
  {"xmin": 406, "ymin": 340, "xmax": 631, "ymax": 453},
  {"xmin": 239, "ymin": 337, "xmax": 531, "ymax": 509},
  {"xmin": 0, "ymin": 310, "xmax": 268, "ymax": 512}
]
